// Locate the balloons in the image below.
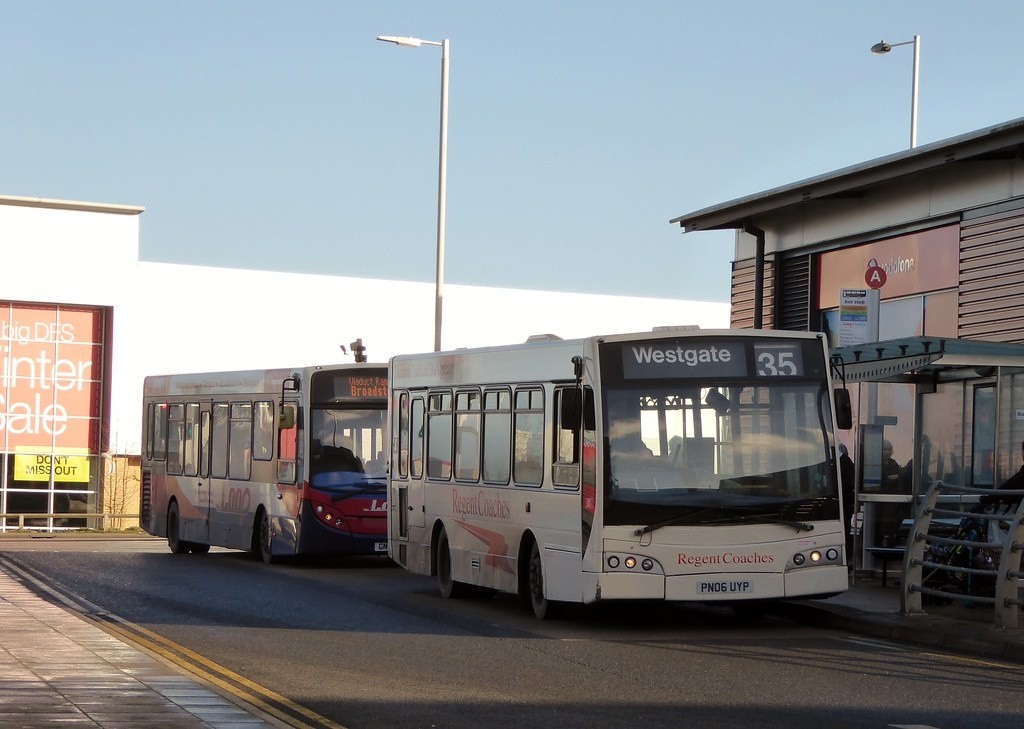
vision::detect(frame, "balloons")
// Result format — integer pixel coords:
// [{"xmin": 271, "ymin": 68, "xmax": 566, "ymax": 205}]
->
[{"xmin": 925, "ymin": 517, "xmax": 1001, "ymax": 592}]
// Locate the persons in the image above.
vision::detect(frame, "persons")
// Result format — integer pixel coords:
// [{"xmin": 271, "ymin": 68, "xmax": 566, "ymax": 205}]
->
[
  {"xmin": 875, "ymin": 439, "xmax": 903, "ymax": 545},
  {"xmin": 605, "ymin": 408, "xmax": 652, "ymax": 460},
  {"xmin": 979, "ymin": 441, "xmax": 1024, "ymax": 510}
]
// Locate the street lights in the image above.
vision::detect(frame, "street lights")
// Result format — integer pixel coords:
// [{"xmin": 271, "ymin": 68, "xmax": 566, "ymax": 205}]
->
[
  {"xmin": 375, "ymin": 35, "xmax": 453, "ymax": 352},
  {"xmin": 869, "ymin": 35, "xmax": 922, "ymax": 148}
]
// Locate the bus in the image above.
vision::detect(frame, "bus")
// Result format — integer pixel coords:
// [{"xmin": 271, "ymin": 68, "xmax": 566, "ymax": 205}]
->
[
  {"xmin": 140, "ymin": 351, "xmax": 390, "ymax": 565},
  {"xmin": 387, "ymin": 326, "xmax": 851, "ymax": 620}
]
